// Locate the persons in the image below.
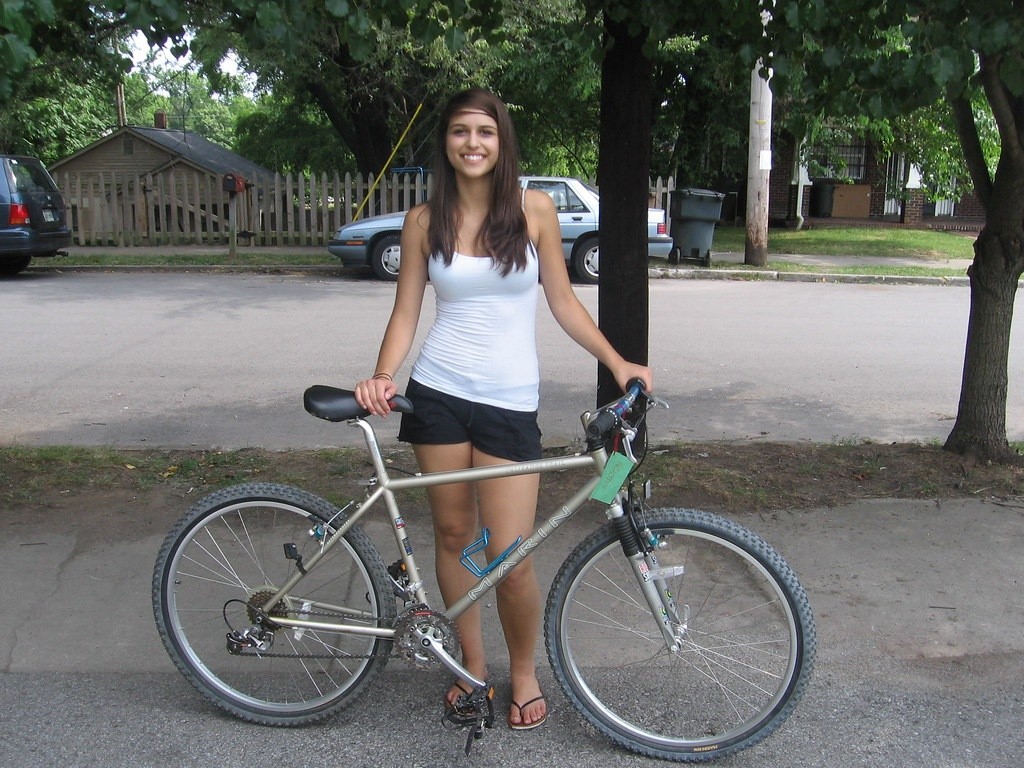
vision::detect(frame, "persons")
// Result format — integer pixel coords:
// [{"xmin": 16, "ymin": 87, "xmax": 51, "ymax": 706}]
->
[{"xmin": 353, "ymin": 87, "xmax": 653, "ymax": 729}]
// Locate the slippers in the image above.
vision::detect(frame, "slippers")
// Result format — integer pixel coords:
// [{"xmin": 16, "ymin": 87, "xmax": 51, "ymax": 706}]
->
[
  {"xmin": 508, "ymin": 681, "xmax": 547, "ymax": 730},
  {"xmin": 443, "ymin": 681, "xmax": 474, "ymax": 711}
]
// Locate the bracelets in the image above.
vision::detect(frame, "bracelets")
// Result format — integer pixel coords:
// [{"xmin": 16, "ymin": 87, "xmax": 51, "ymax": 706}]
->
[{"xmin": 371, "ymin": 373, "xmax": 393, "ymax": 382}]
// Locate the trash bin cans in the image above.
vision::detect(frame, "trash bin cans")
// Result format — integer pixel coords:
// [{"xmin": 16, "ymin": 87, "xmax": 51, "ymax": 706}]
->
[
  {"xmin": 662, "ymin": 188, "xmax": 726, "ymax": 268},
  {"xmin": 809, "ymin": 178, "xmax": 836, "ymax": 218}
]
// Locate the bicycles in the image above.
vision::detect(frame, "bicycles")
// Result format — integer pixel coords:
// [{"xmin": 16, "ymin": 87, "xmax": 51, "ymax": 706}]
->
[{"xmin": 149, "ymin": 375, "xmax": 818, "ymax": 763}]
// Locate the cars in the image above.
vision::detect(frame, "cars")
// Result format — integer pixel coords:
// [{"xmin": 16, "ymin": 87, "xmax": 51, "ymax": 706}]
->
[
  {"xmin": 0, "ymin": 154, "xmax": 74, "ymax": 276},
  {"xmin": 325, "ymin": 176, "xmax": 674, "ymax": 281}
]
